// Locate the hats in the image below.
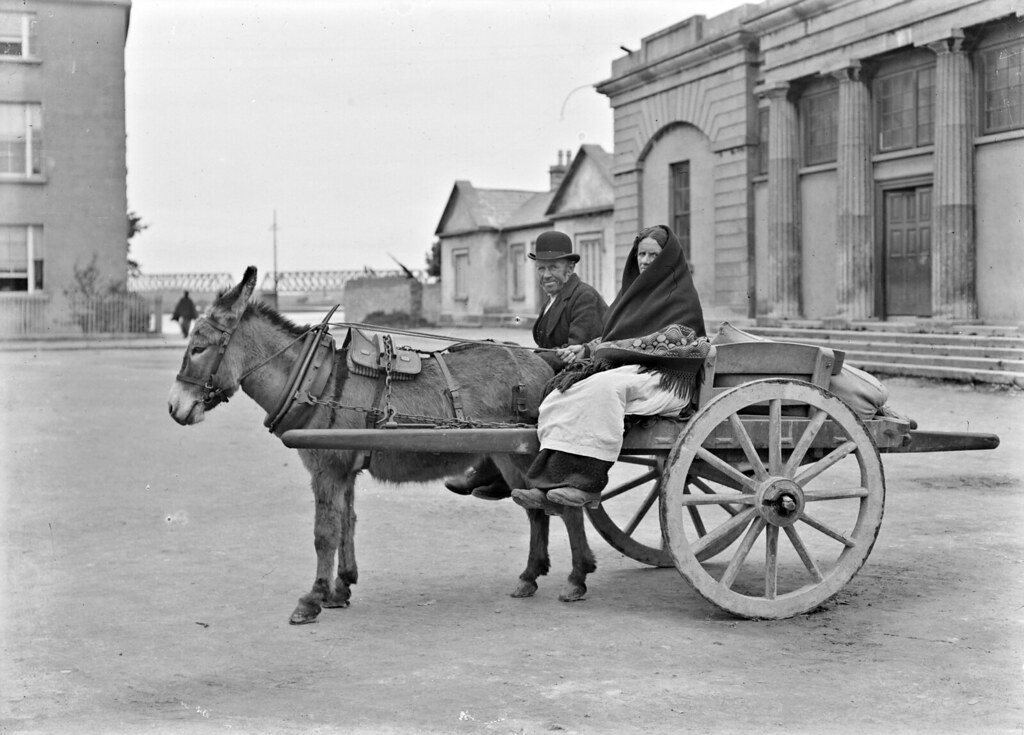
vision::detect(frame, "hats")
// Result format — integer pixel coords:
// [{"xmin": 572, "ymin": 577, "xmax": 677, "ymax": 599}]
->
[{"xmin": 526, "ymin": 230, "xmax": 581, "ymax": 264}]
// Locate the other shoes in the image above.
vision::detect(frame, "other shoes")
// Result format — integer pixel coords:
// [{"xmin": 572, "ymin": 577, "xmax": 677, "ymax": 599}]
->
[
  {"xmin": 470, "ymin": 475, "xmax": 511, "ymax": 501},
  {"xmin": 444, "ymin": 468, "xmax": 500, "ymax": 495}
]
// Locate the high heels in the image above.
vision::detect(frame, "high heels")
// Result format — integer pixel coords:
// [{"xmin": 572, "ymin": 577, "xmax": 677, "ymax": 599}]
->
[
  {"xmin": 546, "ymin": 487, "xmax": 602, "ymax": 510},
  {"xmin": 511, "ymin": 487, "xmax": 565, "ymax": 516}
]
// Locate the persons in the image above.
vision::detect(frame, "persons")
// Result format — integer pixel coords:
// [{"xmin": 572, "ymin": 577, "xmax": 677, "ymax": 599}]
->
[
  {"xmin": 442, "ymin": 232, "xmax": 608, "ymax": 500},
  {"xmin": 171, "ymin": 291, "xmax": 200, "ymax": 337},
  {"xmin": 513, "ymin": 226, "xmax": 707, "ymax": 515}
]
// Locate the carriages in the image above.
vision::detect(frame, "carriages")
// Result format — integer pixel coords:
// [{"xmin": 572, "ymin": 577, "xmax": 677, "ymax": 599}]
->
[{"xmin": 165, "ymin": 264, "xmax": 1001, "ymax": 625}]
